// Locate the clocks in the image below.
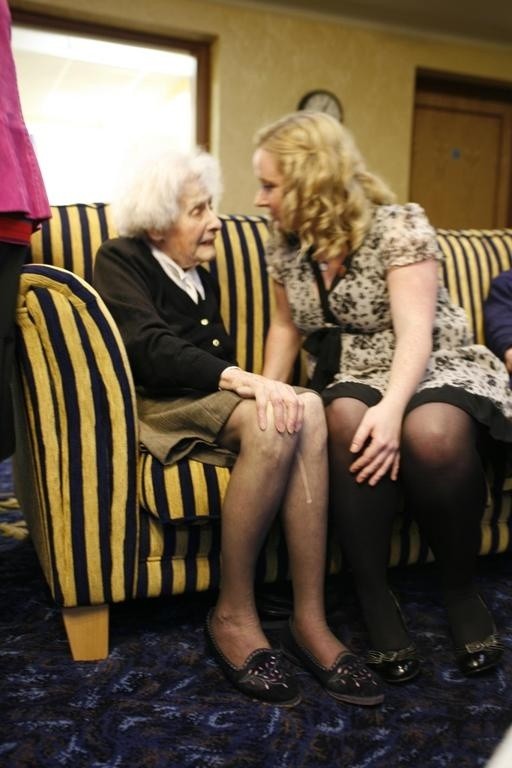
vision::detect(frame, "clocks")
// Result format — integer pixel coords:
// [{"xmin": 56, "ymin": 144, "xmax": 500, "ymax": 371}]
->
[{"xmin": 297, "ymin": 90, "xmax": 344, "ymax": 126}]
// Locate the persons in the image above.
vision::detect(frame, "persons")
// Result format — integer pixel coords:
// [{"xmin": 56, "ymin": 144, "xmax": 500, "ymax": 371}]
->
[
  {"xmin": 250, "ymin": 109, "xmax": 511, "ymax": 685},
  {"xmin": 91, "ymin": 147, "xmax": 387, "ymax": 707},
  {"xmin": 480, "ymin": 268, "xmax": 511, "ymax": 377}
]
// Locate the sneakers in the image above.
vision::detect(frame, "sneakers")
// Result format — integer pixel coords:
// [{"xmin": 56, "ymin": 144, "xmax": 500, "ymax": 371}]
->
[
  {"xmin": 277, "ymin": 610, "xmax": 387, "ymax": 706},
  {"xmin": 203, "ymin": 604, "xmax": 302, "ymax": 710},
  {"xmin": 0, "ymin": 491, "xmax": 31, "ymax": 551}
]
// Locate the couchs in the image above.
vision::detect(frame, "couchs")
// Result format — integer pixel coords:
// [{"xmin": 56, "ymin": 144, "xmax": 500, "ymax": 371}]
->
[{"xmin": 5, "ymin": 201, "xmax": 512, "ymax": 663}]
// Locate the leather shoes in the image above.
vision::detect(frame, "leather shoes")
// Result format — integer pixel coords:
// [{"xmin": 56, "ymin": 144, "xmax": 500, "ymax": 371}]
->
[
  {"xmin": 356, "ymin": 583, "xmax": 421, "ymax": 684},
  {"xmin": 441, "ymin": 585, "xmax": 506, "ymax": 679}
]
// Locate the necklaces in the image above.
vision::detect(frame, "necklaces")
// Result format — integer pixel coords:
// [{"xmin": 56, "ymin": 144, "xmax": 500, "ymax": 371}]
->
[{"xmin": 319, "ymin": 261, "xmax": 328, "ymax": 271}]
